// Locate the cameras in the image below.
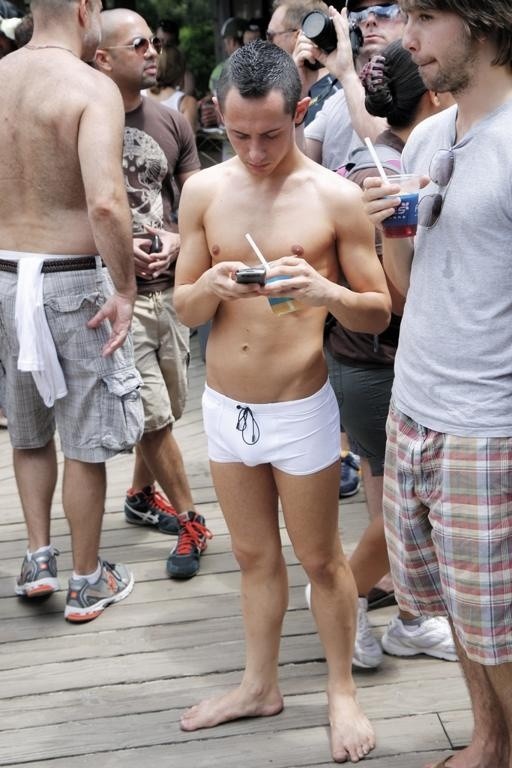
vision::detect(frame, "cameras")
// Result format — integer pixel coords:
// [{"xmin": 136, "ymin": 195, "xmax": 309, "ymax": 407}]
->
[{"xmin": 299, "ymin": 9, "xmax": 365, "ymax": 72}]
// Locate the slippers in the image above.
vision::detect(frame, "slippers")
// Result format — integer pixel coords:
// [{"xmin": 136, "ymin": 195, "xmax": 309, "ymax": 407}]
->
[{"xmin": 368, "ymin": 585, "xmax": 397, "ymax": 610}]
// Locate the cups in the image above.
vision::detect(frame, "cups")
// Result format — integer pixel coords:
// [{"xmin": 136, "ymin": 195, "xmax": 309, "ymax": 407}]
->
[
  {"xmin": 384, "ymin": 173, "xmax": 419, "ymax": 241},
  {"xmin": 258, "ymin": 254, "xmax": 300, "ymax": 319}
]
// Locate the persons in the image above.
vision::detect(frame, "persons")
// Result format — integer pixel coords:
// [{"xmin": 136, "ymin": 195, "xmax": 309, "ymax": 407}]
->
[
  {"xmin": 171, "ymin": 37, "xmax": 395, "ymax": 766},
  {"xmin": 0, "ymin": 1, "xmax": 465, "ymax": 676},
  {"xmin": 359, "ymin": 0, "xmax": 512, "ymax": 768}
]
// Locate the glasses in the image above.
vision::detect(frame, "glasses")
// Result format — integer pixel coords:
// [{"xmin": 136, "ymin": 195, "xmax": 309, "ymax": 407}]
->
[
  {"xmin": 265, "ymin": 28, "xmax": 296, "ymax": 39},
  {"xmin": 345, "ymin": 3, "xmax": 395, "ymax": 25},
  {"xmin": 103, "ymin": 34, "xmax": 164, "ymax": 58},
  {"xmin": 416, "ymin": 148, "xmax": 455, "ymax": 232}
]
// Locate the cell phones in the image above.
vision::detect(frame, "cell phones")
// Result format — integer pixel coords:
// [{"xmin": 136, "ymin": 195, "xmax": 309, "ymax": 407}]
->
[
  {"xmin": 235, "ymin": 268, "xmax": 266, "ymax": 287},
  {"xmin": 148, "ymin": 235, "xmax": 160, "ymax": 255}
]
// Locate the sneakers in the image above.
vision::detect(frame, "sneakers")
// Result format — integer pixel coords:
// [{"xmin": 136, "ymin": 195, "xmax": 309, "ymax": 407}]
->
[
  {"xmin": 64, "ymin": 559, "xmax": 135, "ymax": 623},
  {"xmin": 380, "ymin": 612, "xmax": 460, "ymax": 662},
  {"xmin": 339, "ymin": 451, "xmax": 362, "ymax": 496},
  {"xmin": 13, "ymin": 544, "xmax": 60, "ymax": 599},
  {"xmin": 166, "ymin": 510, "xmax": 213, "ymax": 580},
  {"xmin": 305, "ymin": 582, "xmax": 383, "ymax": 670},
  {"xmin": 124, "ymin": 491, "xmax": 180, "ymax": 535}
]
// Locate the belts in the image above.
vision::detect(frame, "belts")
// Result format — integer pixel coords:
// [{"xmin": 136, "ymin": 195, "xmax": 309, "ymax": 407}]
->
[{"xmin": 1, "ymin": 256, "xmax": 108, "ymax": 275}]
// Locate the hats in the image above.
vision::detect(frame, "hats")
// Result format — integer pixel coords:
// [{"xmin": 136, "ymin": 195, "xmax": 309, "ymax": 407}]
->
[{"xmin": 221, "ymin": 17, "xmax": 250, "ymax": 38}]
